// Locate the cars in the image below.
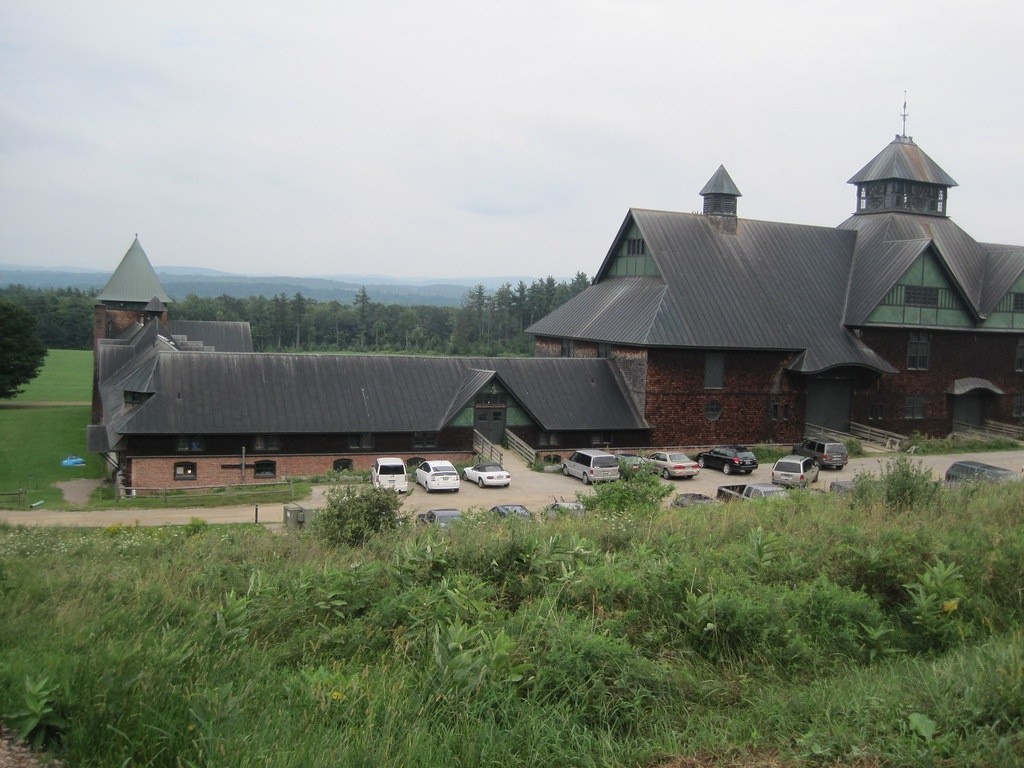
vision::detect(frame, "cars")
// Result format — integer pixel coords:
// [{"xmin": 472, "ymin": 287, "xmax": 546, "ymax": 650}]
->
[
  {"xmin": 462, "ymin": 462, "xmax": 511, "ymax": 489},
  {"xmin": 946, "ymin": 461, "xmax": 1014, "ymax": 484},
  {"xmin": 489, "ymin": 505, "xmax": 530, "ymax": 519},
  {"xmin": 417, "ymin": 508, "xmax": 461, "ymax": 526},
  {"xmin": 615, "ymin": 454, "xmax": 654, "ymax": 476},
  {"xmin": 546, "ymin": 501, "xmax": 585, "ymax": 514},
  {"xmin": 644, "ymin": 451, "xmax": 700, "ymax": 480},
  {"xmin": 415, "ymin": 460, "xmax": 460, "ymax": 493},
  {"xmin": 669, "ymin": 492, "xmax": 721, "ymax": 512}
]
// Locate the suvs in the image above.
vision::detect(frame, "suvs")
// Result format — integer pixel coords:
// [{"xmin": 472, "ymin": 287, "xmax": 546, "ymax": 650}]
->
[
  {"xmin": 561, "ymin": 449, "xmax": 621, "ymax": 485},
  {"xmin": 371, "ymin": 458, "xmax": 409, "ymax": 495},
  {"xmin": 771, "ymin": 455, "xmax": 820, "ymax": 489},
  {"xmin": 791, "ymin": 436, "xmax": 849, "ymax": 471},
  {"xmin": 695, "ymin": 445, "xmax": 759, "ymax": 476}
]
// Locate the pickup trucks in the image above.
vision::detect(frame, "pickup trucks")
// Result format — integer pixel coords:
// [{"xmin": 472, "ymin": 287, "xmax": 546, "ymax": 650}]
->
[{"xmin": 716, "ymin": 482, "xmax": 786, "ymax": 506}]
